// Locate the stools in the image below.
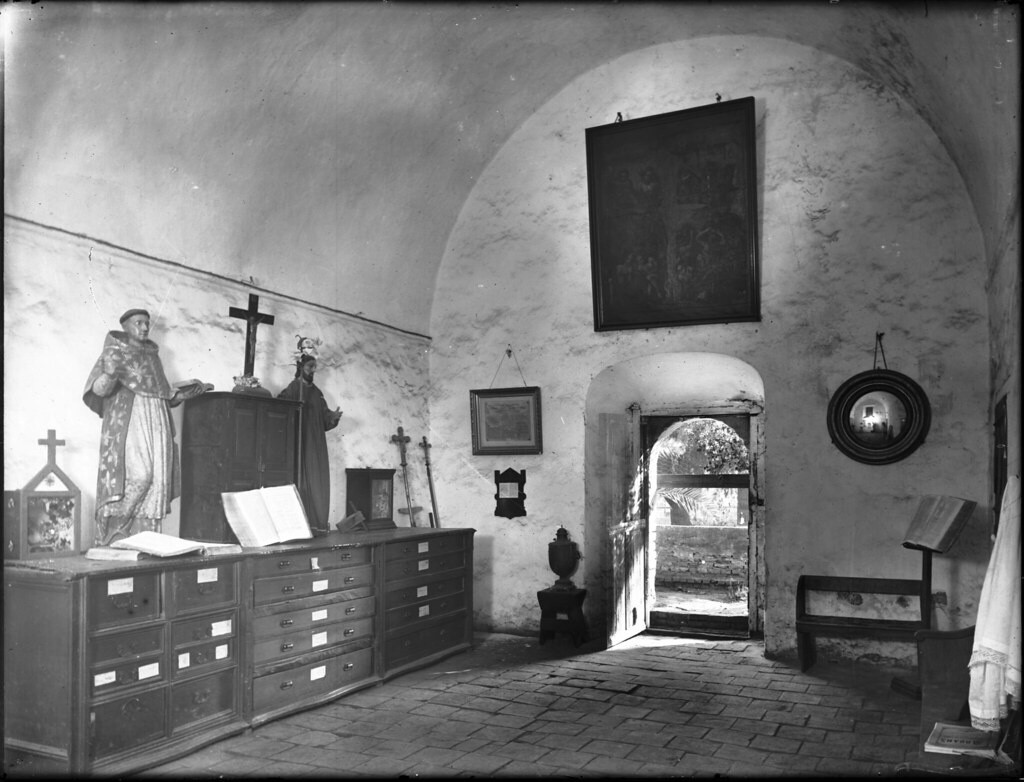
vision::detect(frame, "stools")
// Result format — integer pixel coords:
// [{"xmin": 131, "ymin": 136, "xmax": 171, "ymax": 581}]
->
[{"xmin": 537, "ymin": 588, "xmax": 588, "ymax": 648}]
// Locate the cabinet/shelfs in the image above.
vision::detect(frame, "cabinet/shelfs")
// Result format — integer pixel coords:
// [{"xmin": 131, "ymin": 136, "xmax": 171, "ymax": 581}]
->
[
  {"xmin": 178, "ymin": 391, "xmax": 305, "ymax": 545},
  {"xmin": 5, "ymin": 526, "xmax": 477, "ymax": 776}
]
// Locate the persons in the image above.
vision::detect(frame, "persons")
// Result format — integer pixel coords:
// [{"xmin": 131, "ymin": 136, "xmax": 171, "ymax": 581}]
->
[
  {"xmin": 82, "ymin": 308, "xmax": 202, "ymax": 547},
  {"xmin": 277, "ymin": 355, "xmax": 343, "ymax": 537}
]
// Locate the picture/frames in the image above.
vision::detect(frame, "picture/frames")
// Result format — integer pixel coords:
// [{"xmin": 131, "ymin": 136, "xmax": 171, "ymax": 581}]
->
[
  {"xmin": 585, "ymin": 97, "xmax": 762, "ymax": 333},
  {"xmin": 469, "ymin": 386, "xmax": 544, "ymax": 455}
]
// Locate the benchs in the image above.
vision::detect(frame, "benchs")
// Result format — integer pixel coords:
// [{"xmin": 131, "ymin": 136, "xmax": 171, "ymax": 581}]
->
[
  {"xmin": 893, "ymin": 625, "xmax": 1023, "ymax": 777},
  {"xmin": 794, "ymin": 575, "xmax": 928, "ymax": 673}
]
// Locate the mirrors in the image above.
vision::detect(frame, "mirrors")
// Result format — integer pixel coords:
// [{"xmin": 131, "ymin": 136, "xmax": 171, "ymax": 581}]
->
[{"xmin": 826, "ymin": 367, "xmax": 932, "ymax": 465}]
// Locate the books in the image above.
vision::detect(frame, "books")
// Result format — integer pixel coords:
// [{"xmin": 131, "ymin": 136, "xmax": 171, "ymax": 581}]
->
[
  {"xmin": 172, "ymin": 378, "xmax": 214, "ymax": 392},
  {"xmin": 220, "ymin": 483, "xmax": 314, "ymax": 548},
  {"xmin": 83, "ymin": 531, "xmax": 243, "ymax": 562},
  {"xmin": 900, "ymin": 494, "xmax": 978, "ymax": 554},
  {"xmin": 923, "ymin": 712, "xmax": 1020, "ymax": 766}
]
